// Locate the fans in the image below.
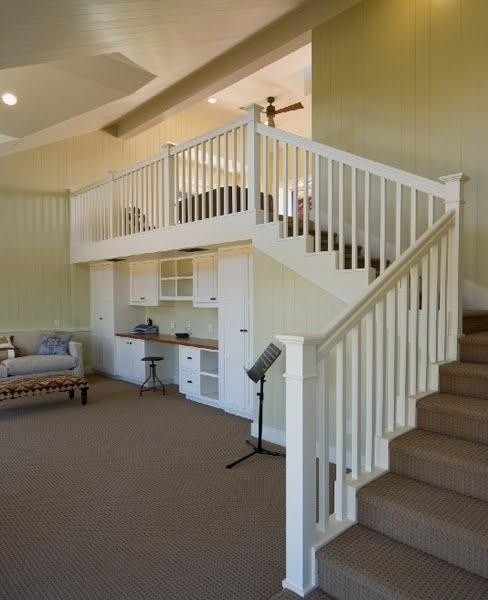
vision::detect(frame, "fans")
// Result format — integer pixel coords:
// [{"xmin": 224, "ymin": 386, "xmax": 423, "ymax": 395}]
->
[{"xmin": 240, "ymin": 96, "xmax": 304, "ymax": 128}]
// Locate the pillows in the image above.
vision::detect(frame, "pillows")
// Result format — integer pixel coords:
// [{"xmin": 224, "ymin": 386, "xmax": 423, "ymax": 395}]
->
[{"xmin": 38, "ymin": 333, "xmax": 72, "ymax": 356}]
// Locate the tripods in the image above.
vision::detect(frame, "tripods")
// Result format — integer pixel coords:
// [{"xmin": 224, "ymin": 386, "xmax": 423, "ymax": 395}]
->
[{"xmin": 225, "ymin": 375, "xmax": 286, "ymax": 469}]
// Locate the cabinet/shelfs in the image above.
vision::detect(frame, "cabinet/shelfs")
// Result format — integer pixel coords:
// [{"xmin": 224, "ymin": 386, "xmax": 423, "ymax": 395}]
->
[
  {"xmin": 159, "ymin": 256, "xmax": 193, "ymax": 301},
  {"xmin": 115, "ymin": 336, "xmax": 146, "ymax": 388},
  {"xmin": 129, "ymin": 259, "xmax": 159, "ymax": 307},
  {"xmin": 89, "ymin": 261, "xmax": 145, "ymax": 382},
  {"xmin": 193, "ymin": 253, "xmax": 218, "ymax": 308},
  {"xmin": 218, "ymin": 244, "xmax": 285, "ymax": 422},
  {"xmin": 179, "ymin": 344, "xmax": 219, "ymax": 403}
]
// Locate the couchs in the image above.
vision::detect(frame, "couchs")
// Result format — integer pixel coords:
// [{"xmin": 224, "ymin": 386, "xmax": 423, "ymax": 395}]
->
[{"xmin": 1, "ymin": 331, "xmax": 84, "ymax": 377}]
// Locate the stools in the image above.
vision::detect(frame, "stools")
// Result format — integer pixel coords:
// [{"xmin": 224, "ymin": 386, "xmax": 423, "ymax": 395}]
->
[{"xmin": 140, "ymin": 356, "xmax": 165, "ymax": 397}]
